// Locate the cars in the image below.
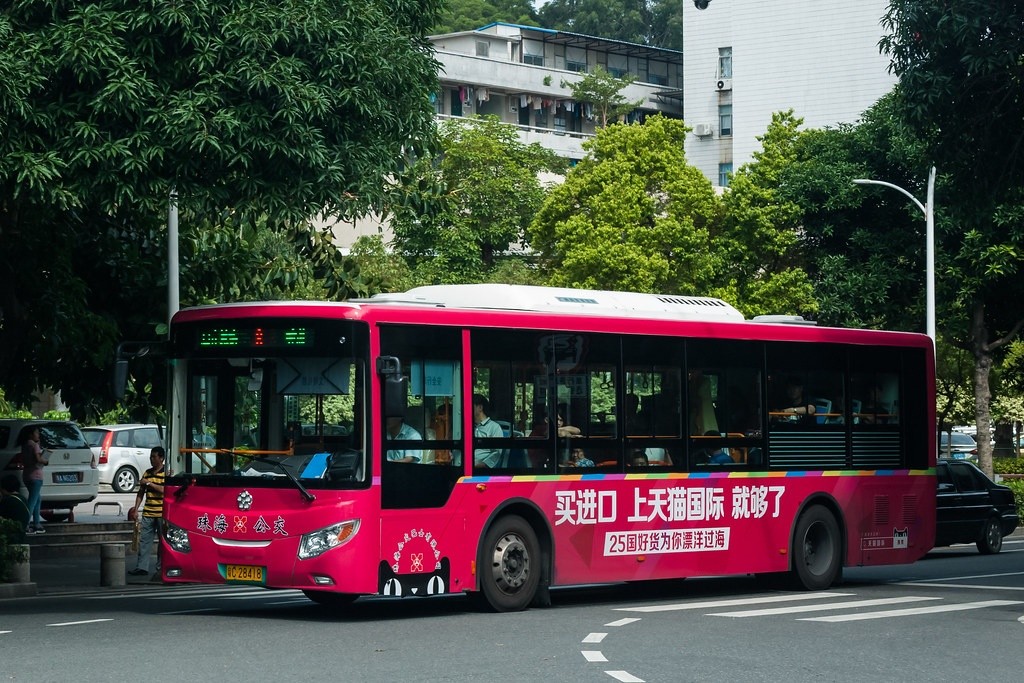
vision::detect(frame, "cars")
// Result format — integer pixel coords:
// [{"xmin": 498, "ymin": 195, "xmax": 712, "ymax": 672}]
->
[
  {"xmin": 955, "ymin": 426, "xmax": 1024, "ymax": 451},
  {"xmin": 81, "ymin": 424, "xmax": 218, "ymax": 493},
  {"xmin": 935, "ymin": 458, "xmax": 1023, "ymax": 549},
  {"xmin": 936, "ymin": 431, "xmax": 979, "ymax": 463}
]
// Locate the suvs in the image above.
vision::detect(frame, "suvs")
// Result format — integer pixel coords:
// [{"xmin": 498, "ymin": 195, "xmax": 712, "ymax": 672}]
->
[{"xmin": 0, "ymin": 418, "xmax": 100, "ymax": 520}]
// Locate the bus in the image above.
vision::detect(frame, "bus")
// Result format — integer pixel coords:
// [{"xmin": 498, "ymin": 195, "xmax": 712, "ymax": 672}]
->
[{"xmin": 165, "ymin": 285, "xmax": 937, "ymax": 620}]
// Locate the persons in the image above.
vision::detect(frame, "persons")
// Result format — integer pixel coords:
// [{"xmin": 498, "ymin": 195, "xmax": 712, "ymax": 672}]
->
[
  {"xmin": 384, "ymin": 411, "xmax": 423, "ymax": 463},
  {"xmin": 438, "ymin": 393, "xmax": 504, "ymax": 467},
  {"xmin": 847, "ymin": 379, "xmax": 889, "ymax": 424},
  {"xmin": 128, "ymin": 446, "xmax": 167, "ymax": 576},
  {"xmin": 22, "ymin": 426, "xmax": 50, "ymax": 535},
  {"xmin": 773, "ymin": 379, "xmax": 818, "ymax": 427},
  {"xmin": 526, "ymin": 409, "xmax": 596, "ymax": 467},
  {"xmin": 703, "ymin": 430, "xmax": 734, "ymax": 465},
  {"xmin": 627, "ymin": 451, "xmax": 653, "ymax": 468},
  {"xmin": 548, "ymin": 375, "xmax": 719, "ymax": 436}
]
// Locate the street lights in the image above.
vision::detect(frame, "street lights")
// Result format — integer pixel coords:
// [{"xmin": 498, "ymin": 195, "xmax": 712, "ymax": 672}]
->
[{"xmin": 852, "ymin": 165, "xmax": 939, "ymax": 360}]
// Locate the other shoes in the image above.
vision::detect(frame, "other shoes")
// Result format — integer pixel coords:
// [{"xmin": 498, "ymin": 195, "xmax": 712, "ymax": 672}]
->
[
  {"xmin": 33, "ymin": 526, "xmax": 46, "ymax": 533},
  {"xmin": 25, "ymin": 528, "xmax": 36, "ymax": 535},
  {"xmin": 127, "ymin": 567, "xmax": 148, "ymax": 575}
]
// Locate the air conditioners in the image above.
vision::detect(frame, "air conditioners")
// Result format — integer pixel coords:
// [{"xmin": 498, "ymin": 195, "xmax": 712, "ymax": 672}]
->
[
  {"xmin": 714, "ymin": 79, "xmax": 732, "ymax": 90},
  {"xmin": 693, "ymin": 124, "xmax": 713, "ymax": 137}
]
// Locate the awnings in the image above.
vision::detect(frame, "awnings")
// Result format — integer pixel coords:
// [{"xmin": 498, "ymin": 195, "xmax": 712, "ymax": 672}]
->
[{"xmin": 652, "ymin": 88, "xmax": 683, "ymax": 106}]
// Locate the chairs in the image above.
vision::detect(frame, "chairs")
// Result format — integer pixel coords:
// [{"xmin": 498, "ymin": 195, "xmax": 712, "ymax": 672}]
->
[
  {"xmin": 815, "ymin": 399, "xmax": 861, "ymax": 424},
  {"xmin": 406, "ymin": 409, "xmax": 533, "ymax": 475}
]
